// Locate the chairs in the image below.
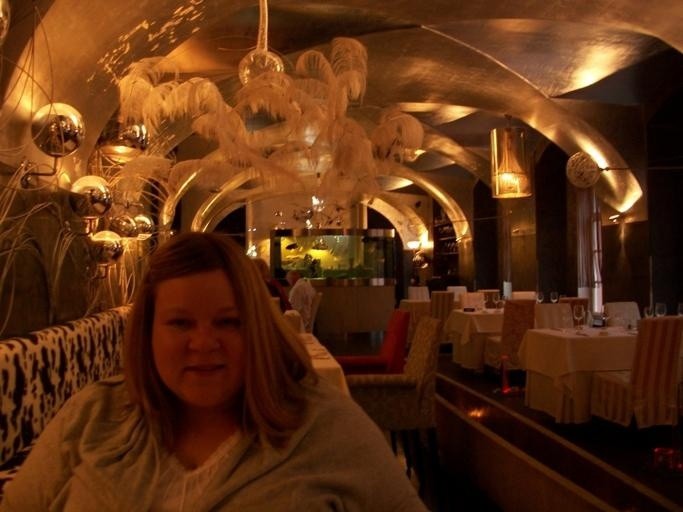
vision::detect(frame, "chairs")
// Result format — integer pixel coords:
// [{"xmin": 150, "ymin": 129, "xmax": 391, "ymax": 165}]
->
[{"xmin": 331, "ymin": 310, "xmax": 444, "ymax": 458}]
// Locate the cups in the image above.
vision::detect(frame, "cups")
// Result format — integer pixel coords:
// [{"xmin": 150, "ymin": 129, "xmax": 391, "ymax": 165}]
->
[
  {"xmin": 551, "ymin": 292, "xmax": 558, "ymax": 303},
  {"xmin": 644, "ymin": 303, "xmax": 667, "ymax": 318},
  {"xmin": 536, "ymin": 291, "xmax": 544, "ymax": 303}
]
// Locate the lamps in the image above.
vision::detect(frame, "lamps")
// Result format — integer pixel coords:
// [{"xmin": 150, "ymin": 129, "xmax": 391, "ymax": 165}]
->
[
  {"xmin": 406, "ymin": 238, "xmax": 434, "ymax": 269},
  {"xmin": 489, "ymin": 112, "xmax": 536, "ymax": 199}
]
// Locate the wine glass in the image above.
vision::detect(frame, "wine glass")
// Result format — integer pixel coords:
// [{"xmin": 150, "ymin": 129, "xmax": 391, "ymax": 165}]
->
[
  {"xmin": 574, "ymin": 304, "xmax": 584, "ymax": 330},
  {"xmin": 492, "ymin": 293, "xmax": 500, "ymax": 310}
]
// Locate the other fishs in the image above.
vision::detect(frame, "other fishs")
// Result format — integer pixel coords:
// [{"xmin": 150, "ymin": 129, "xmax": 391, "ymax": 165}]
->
[
  {"xmin": 285, "ymin": 242, "xmax": 299, "ymax": 251},
  {"xmin": 312, "ymin": 241, "xmax": 328, "ymax": 250}
]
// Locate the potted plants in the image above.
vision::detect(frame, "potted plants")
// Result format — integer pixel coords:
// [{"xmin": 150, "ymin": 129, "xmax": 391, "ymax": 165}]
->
[{"xmin": 296, "ymin": 248, "xmax": 384, "ymax": 286}]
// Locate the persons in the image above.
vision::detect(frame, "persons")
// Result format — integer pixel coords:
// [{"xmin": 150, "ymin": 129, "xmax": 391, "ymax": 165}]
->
[
  {"xmin": 0, "ymin": 230, "xmax": 432, "ymax": 512},
  {"xmin": 284, "ymin": 269, "xmax": 317, "ymax": 334},
  {"xmin": 251, "ymin": 259, "xmax": 293, "ymax": 314}
]
